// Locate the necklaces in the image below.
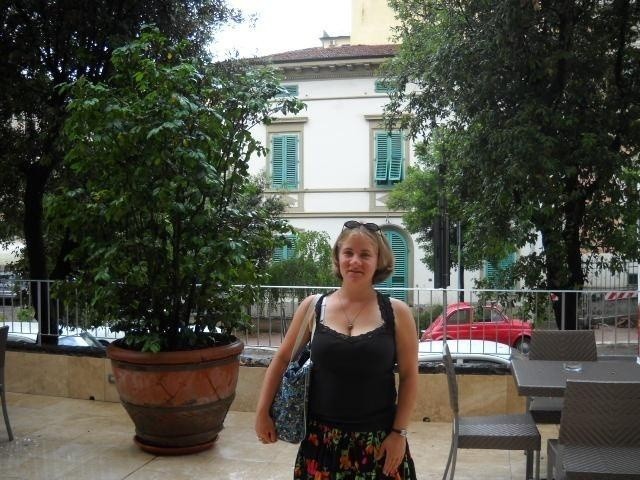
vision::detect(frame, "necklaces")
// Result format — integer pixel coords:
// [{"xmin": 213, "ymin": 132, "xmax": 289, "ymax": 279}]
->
[{"xmin": 336, "ymin": 289, "xmax": 372, "ymax": 330}]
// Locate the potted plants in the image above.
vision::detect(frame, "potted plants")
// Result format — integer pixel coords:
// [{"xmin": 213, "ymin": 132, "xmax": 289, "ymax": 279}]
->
[{"xmin": 44, "ymin": 30, "xmax": 308, "ymax": 456}]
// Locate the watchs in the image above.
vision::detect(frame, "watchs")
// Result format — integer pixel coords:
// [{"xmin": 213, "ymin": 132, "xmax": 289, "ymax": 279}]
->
[{"xmin": 392, "ymin": 428, "xmax": 407, "ymax": 437}]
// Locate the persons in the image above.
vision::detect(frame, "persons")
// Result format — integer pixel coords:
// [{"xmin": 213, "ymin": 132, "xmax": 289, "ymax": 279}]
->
[{"xmin": 255, "ymin": 220, "xmax": 417, "ymax": 480}]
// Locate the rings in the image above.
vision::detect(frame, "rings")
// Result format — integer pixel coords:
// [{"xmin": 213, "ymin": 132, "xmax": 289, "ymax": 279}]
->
[{"xmin": 258, "ymin": 438, "xmax": 262, "ymax": 440}]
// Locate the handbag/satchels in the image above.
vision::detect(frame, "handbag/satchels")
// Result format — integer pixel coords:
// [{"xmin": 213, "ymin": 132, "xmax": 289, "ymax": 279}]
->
[{"xmin": 268, "ymin": 343, "xmax": 314, "ymax": 445}]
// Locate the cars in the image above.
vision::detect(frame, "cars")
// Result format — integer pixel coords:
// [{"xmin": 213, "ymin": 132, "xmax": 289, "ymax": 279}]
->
[
  {"xmin": 419, "ymin": 298, "xmax": 536, "ymax": 356},
  {"xmin": 413, "ymin": 339, "xmax": 533, "ymax": 374},
  {"xmin": 0, "ymin": 270, "xmax": 31, "ymax": 305},
  {"xmin": 0, "ymin": 317, "xmax": 225, "ymax": 351}
]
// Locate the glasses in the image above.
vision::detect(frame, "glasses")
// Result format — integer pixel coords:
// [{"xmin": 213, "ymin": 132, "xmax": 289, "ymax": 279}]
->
[{"xmin": 342, "ymin": 220, "xmax": 384, "ymax": 239}]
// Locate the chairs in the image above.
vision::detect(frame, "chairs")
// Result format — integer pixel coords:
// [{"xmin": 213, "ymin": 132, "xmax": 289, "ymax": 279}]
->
[{"xmin": 435, "ymin": 330, "xmax": 640, "ymax": 479}]
